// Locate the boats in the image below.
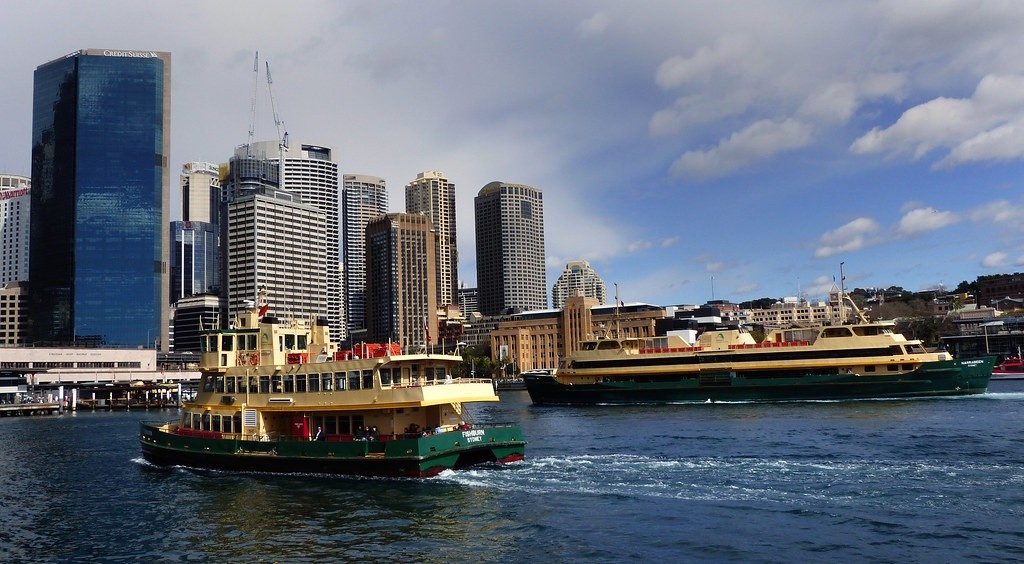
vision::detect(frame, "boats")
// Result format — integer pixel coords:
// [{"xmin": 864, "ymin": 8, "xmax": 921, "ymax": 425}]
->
[
  {"xmin": 519, "ymin": 261, "xmax": 998, "ymax": 401},
  {"xmin": 990, "ymin": 345, "xmax": 1024, "ymax": 380},
  {"xmin": 140, "ymin": 284, "xmax": 528, "ymax": 478}
]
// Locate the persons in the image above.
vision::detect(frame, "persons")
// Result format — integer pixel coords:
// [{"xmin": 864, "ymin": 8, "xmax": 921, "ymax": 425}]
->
[
  {"xmin": 313, "ymin": 426, "xmax": 325, "ymax": 441},
  {"xmin": 353, "ymin": 426, "xmax": 381, "ymax": 442}
]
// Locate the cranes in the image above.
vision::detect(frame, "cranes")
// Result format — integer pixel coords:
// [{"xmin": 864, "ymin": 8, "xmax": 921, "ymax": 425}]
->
[
  {"xmin": 243, "ymin": 51, "xmax": 258, "ymax": 159},
  {"xmin": 266, "ymin": 62, "xmax": 290, "ymax": 190}
]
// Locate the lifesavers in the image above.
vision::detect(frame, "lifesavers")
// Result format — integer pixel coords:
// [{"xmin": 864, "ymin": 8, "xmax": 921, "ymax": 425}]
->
[{"xmin": 250, "ymin": 354, "xmax": 258, "ymax": 366}]
